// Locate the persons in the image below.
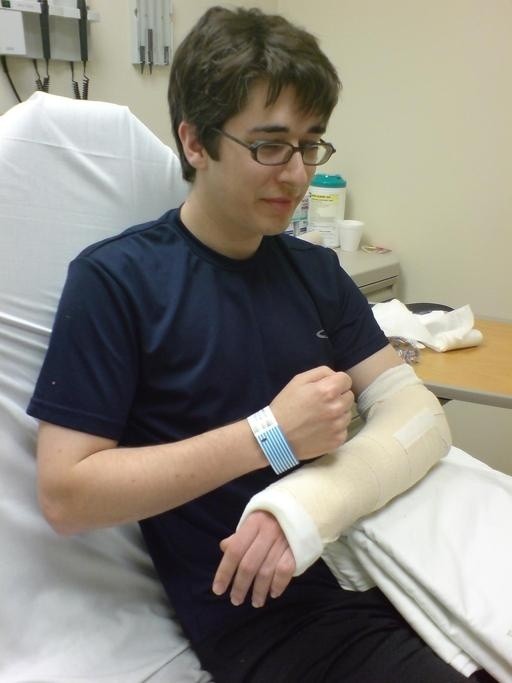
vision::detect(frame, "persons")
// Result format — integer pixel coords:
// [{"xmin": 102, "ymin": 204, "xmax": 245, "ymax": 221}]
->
[{"xmin": 27, "ymin": 7, "xmax": 505, "ymax": 683}]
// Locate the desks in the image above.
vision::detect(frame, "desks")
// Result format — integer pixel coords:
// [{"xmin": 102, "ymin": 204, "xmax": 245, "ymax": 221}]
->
[
  {"xmin": 334, "ymin": 247, "xmax": 401, "ymax": 302},
  {"xmin": 413, "ymin": 319, "xmax": 512, "ymax": 410}
]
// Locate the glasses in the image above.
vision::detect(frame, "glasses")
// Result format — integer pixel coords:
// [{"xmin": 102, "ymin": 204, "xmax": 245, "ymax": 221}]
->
[{"xmin": 211, "ymin": 126, "xmax": 336, "ymax": 166}]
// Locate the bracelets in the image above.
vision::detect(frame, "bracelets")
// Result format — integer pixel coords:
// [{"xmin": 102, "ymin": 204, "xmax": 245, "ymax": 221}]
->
[{"xmin": 248, "ymin": 405, "xmax": 300, "ymax": 477}]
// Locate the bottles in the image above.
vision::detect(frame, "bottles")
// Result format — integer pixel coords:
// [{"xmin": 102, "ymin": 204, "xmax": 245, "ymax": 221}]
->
[{"xmin": 308, "ymin": 174, "xmax": 347, "ymax": 246}]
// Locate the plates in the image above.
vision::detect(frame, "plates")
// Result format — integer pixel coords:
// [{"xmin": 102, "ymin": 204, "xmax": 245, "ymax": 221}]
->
[{"xmin": 403, "ymin": 302, "xmax": 453, "ymax": 314}]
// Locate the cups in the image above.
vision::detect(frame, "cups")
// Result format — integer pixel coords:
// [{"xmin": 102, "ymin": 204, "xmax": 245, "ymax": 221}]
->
[{"xmin": 339, "ymin": 218, "xmax": 363, "ymax": 251}]
